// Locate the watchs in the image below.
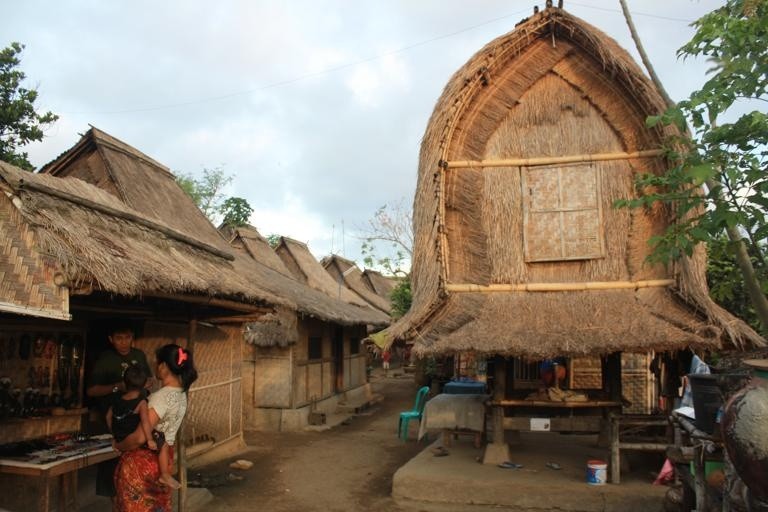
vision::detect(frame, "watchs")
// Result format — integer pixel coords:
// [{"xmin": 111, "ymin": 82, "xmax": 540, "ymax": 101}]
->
[{"xmin": 112, "ymin": 383, "xmax": 119, "ymax": 393}]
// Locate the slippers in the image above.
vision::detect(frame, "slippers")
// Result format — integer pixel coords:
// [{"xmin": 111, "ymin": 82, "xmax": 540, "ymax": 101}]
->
[
  {"xmin": 498, "ymin": 461, "xmax": 523, "ymax": 468},
  {"xmin": 546, "ymin": 461, "xmax": 560, "ymax": 470}
]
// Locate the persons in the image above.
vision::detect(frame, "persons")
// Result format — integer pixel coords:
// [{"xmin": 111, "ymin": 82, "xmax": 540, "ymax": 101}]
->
[
  {"xmin": 106, "ymin": 363, "xmax": 181, "ymax": 490},
  {"xmin": 382, "ymin": 350, "xmax": 391, "ymax": 373},
  {"xmin": 86, "ymin": 320, "xmax": 155, "ymax": 496},
  {"xmin": 111, "ymin": 343, "xmax": 197, "ymax": 512}
]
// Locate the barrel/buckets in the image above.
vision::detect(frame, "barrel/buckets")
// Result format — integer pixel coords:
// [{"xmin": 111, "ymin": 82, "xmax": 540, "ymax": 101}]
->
[{"xmin": 585, "ymin": 460, "xmax": 608, "ymax": 486}]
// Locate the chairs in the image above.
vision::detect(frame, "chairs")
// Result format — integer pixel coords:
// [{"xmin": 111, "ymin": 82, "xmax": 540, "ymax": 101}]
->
[{"xmin": 397, "ymin": 386, "xmax": 430, "ymax": 442}]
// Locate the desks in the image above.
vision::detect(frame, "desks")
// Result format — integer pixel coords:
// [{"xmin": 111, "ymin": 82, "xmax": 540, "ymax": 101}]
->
[
  {"xmin": 605, "ymin": 406, "xmax": 672, "ymax": 485},
  {"xmin": 482, "ymin": 393, "xmax": 629, "ymax": 450},
  {"xmin": 0, "ymin": 447, "xmax": 122, "ymax": 512},
  {"xmin": 416, "ymin": 393, "xmax": 486, "ymax": 449}
]
